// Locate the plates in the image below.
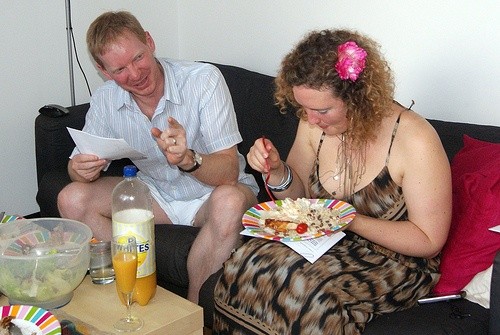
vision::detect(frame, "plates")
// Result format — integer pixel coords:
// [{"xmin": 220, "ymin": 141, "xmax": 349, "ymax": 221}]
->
[
  {"xmin": 242, "ymin": 199, "xmax": 356, "ymax": 241},
  {"xmin": 0, "ymin": 305, "xmax": 61, "ymax": 335}
]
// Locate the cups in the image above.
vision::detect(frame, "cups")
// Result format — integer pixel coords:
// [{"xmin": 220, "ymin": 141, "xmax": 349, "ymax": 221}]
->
[{"xmin": 89, "ymin": 241, "xmax": 114, "ymax": 285}]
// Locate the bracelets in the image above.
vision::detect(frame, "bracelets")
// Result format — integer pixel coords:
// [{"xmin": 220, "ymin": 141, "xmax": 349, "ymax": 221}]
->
[{"xmin": 262, "ymin": 160, "xmax": 293, "ymax": 192}]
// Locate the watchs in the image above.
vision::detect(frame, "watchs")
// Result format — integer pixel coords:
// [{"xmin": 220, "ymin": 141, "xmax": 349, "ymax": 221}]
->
[{"xmin": 177, "ymin": 148, "xmax": 203, "ymax": 173}]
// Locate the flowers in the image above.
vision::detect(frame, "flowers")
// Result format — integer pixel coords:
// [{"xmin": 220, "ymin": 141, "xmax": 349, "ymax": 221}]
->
[{"xmin": 335, "ymin": 41, "xmax": 367, "ymax": 83}]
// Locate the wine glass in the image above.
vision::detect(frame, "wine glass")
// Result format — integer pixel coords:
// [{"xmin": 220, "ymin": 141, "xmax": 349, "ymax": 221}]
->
[{"xmin": 110, "ymin": 233, "xmax": 143, "ymax": 333}]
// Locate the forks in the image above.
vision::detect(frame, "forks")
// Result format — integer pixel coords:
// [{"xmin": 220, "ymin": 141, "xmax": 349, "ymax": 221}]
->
[{"xmin": 262, "ymin": 135, "xmax": 276, "ymax": 204}]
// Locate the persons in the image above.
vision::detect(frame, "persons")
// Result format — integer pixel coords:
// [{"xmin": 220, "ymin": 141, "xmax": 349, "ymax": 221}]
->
[
  {"xmin": 55, "ymin": 13, "xmax": 260, "ymax": 305},
  {"xmin": 211, "ymin": 26, "xmax": 453, "ymax": 335}
]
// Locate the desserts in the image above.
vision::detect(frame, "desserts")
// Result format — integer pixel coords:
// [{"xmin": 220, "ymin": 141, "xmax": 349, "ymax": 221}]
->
[{"xmin": 265, "ymin": 197, "xmax": 340, "ymax": 235}]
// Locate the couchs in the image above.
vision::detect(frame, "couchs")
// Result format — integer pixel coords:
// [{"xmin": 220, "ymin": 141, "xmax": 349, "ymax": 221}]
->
[{"xmin": 35, "ymin": 62, "xmax": 500, "ymax": 335}]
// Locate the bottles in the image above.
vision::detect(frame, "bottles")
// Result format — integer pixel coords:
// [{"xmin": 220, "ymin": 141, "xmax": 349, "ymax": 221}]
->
[{"xmin": 111, "ymin": 165, "xmax": 157, "ymax": 306}]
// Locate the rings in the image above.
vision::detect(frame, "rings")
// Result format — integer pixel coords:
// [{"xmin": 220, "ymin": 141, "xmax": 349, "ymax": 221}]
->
[{"xmin": 173, "ymin": 137, "xmax": 176, "ymax": 145}]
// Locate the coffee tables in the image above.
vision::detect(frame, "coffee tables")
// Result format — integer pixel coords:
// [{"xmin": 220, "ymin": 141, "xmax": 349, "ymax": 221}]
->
[{"xmin": 0, "ymin": 224, "xmax": 204, "ymax": 335}]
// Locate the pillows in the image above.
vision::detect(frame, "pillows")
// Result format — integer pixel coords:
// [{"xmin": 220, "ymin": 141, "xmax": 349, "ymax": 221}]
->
[{"xmin": 429, "ymin": 134, "xmax": 500, "ymax": 297}]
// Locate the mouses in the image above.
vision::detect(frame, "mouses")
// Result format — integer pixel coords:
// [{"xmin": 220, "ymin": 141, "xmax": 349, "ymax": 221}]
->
[{"xmin": 39, "ymin": 104, "xmax": 69, "ymax": 117}]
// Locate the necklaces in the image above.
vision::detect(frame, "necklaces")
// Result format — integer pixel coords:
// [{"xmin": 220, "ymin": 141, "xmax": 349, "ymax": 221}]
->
[{"xmin": 333, "ymin": 152, "xmax": 357, "ymax": 181}]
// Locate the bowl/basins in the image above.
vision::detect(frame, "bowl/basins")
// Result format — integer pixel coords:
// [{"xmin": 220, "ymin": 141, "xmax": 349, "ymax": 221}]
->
[{"xmin": 0, "ymin": 218, "xmax": 93, "ymax": 311}]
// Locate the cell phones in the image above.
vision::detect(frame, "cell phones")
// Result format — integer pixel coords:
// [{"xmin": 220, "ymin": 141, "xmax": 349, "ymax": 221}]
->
[{"xmin": 418, "ymin": 291, "xmax": 466, "ymax": 305}]
